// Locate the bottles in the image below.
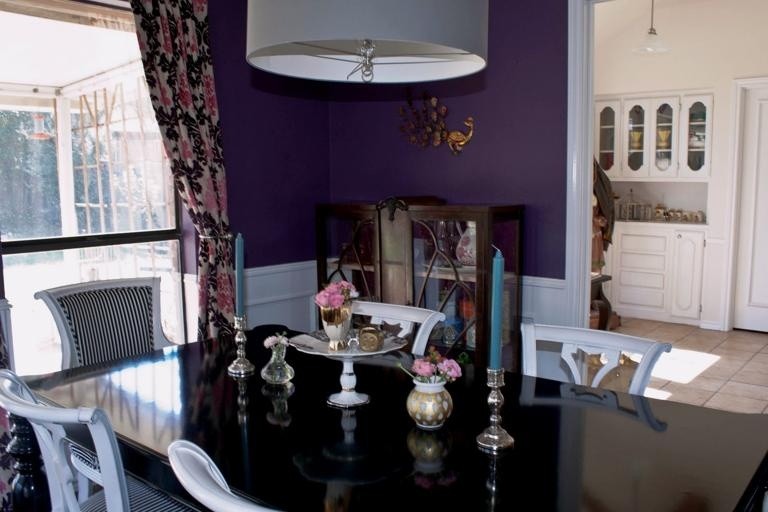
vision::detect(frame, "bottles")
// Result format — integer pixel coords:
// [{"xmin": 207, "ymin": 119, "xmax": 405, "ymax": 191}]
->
[{"xmin": 454, "ymin": 222, "xmax": 475, "ymax": 268}]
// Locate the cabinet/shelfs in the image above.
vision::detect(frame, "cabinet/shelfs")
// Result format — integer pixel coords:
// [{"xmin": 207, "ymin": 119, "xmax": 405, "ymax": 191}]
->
[
  {"xmin": 314, "ymin": 195, "xmax": 527, "ymax": 373},
  {"xmin": 594, "ymin": 97, "xmax": 649, "ymax": 183},
  {"xmin": 648, "ymin": 94, "xmax": 713, "ymax": 182},
  {"xmin": 601, "ymin": 222, "xmax": 668, "ymax": 323},
  {"xmin": 668, "ymin": 225, "xmax": 705, "ymax": 325}
]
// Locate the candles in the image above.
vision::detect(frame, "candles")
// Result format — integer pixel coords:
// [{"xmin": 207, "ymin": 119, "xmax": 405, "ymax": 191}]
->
[
  {"xmin": 235, "ymin": 231, "xmax": 245, "ymax": 316},
  {"xmin": 488, "ymin": 243, "xmax": 506, "ymax": 372}
]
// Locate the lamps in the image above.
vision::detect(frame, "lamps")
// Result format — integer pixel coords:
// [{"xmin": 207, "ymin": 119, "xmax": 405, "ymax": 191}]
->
[
  {"xmin": 642, "ymin": 0, "xmax": 658, "ymax": 52},
  {"xmin": 243, "ymin": 0, "xmax": 490, "ymax": 85}
]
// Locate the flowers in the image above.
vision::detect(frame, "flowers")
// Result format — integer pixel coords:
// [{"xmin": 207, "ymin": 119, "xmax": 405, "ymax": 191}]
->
[
  {"xmin": 311, "ymin": 281, "xmax": 359, "ymax": 309},
  {"xmin": 393, "ymin": 345, "xmax": 468, "ymax": 383},
  {"xmin": 266, "ymin": 387, "xmax": 291, "ymax": 426},
  {"xmin": 407, "ymin": 463, "xmax": 471, "ymax": 490},
  {"xmin": 262, "ymin": 331, "xmax": 292, "ymax": 381}
]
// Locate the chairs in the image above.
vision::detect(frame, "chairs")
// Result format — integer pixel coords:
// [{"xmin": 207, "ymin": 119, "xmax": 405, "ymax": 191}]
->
[
  {"xmin": 166, "ymin": 438, "xmax": 282, "ymax": 511},
  {"xmin": 0, "ymin": 367, "xmax": 200, "ymax": 511},
  {"xmin": 32, "ymin": 274, "xmax": 179, "ymax": 372},
  {"xmin": 520, "ymin": 375, "xmax": 672, "ymax": 434},
  {"xmin": 519, "ymin": 320, "xmax": 672, "ymax": 396},
  {"xmin": 349, "ymin": 298, "xmax": 446, "ymax": 357}
]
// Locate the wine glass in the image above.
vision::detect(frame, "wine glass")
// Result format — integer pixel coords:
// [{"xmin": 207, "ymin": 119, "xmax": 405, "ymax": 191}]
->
[{"xmin": 287, "ymin": 325, "xmax": 408, "ymax": 408}]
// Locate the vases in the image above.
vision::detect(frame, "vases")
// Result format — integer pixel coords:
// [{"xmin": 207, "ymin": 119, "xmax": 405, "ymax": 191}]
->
[
  {"xmin": 405, "ymin": 429, "xmax": 449, "ymax": 475},
  {"xmin": 262, "ymin": 384, "xmax": 296, "ymax": 419},
  {"xmin": 404, "ymin": 375, "xmax": 454, "ymax": 431},
  {"xmin": 260, "ymin": 345, "xmax": 296, "ymax": 384},
  {"xmin": 325, "ymin": 482, "xmax": 352, "ymax": 512},
  {"xmin": 319, "ymin": 304, "xmax": 352, "ymax": 352}
]
